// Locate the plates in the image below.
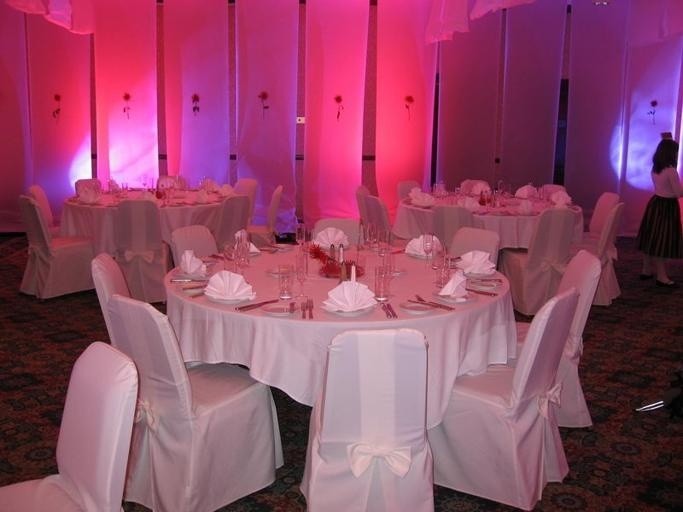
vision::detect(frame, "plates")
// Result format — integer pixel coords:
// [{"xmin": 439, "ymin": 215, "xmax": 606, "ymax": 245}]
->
[
  {"xmin": 257, "ymin": 302, "xmax": 301, "ymax": 316},
  {"xmin": 327, "ymin": 303, "xmax": 374, "ymax": 317},
  {"xmin": 173, "ymin": 239, "xmax": 501, "ymax": 288},
  {"xmin": 206, "ymin": 293, "xmax": 252, "ymax": 304},
  {"xmin": 432, "ymin": 290, "xmax": 479, "ymax": 305}
]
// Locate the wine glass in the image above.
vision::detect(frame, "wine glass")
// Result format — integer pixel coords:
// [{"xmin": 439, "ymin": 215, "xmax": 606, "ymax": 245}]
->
[
  {"xmin": 423, "ymin": 230, "xmax": 446, "ymax": 287},
  {"xmin": 296, "ymin": 224, "xmax": 315, "ymax": 249},
  {"xmin": 278, "ymin": 252, "xmax": 308, "ymax": 299},
  {"xmin": 380, "ymin": 248, "xmax": 397, "ymax": 299}
]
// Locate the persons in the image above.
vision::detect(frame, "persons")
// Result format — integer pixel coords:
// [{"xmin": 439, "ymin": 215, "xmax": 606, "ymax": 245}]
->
[{"xmin": 635, "ymin": 138, "xmax": 682, "ymax": 287}]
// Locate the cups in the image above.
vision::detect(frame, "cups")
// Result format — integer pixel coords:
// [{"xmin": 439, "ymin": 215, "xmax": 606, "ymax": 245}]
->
[
  {"xmin": 224, "ymin": 232, "xmax": 252, "ymax": 274},
  {"xmin": 436, "ymin": 256, "xmax": 450, "ymax": 288},
  {"xmin": 365, "ymin": 222, "xmax": 391, "ymax": 257},
  {"xmin": 376, "ymin": 264, "xmax": 392, "ymax": 303}
]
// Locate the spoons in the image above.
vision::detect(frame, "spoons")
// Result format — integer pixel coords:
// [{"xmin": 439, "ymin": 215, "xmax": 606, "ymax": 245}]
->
[{"xmin": 260, "ymin": 248, "xmax": 278, "ymax": 254}]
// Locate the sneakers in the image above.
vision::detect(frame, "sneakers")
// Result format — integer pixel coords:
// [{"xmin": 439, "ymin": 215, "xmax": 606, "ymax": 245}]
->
[
  {"xmin": 639, "ymin": 274, "xmax": 654, "ymax": 280},
  {"xmin": 655, "ymin": 279, "xmax": 678, "ymax": 288}
]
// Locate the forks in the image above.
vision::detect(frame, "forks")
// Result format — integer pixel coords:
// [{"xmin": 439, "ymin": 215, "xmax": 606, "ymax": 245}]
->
[
  {"xmin": 302, "ymin": 298, "xmax": 315, "ymax": 320},
  {"xmin": 415, "ymin": 293, "xmax": 455, "ymax": 311}
]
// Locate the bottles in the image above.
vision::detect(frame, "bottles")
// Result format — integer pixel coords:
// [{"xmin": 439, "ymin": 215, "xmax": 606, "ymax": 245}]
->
[
  {"xmin": 330, "ymin": 244, "xmax": 344, "ymax": 263},
  {"xmin": 340, "ymin": 262, "xmax": 356, "ymax": 282}
]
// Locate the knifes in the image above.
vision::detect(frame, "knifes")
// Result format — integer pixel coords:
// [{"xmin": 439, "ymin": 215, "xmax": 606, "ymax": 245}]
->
[{"xmin": 407, "ymin": 299, "xmax": 439, "ymax": 309}]
